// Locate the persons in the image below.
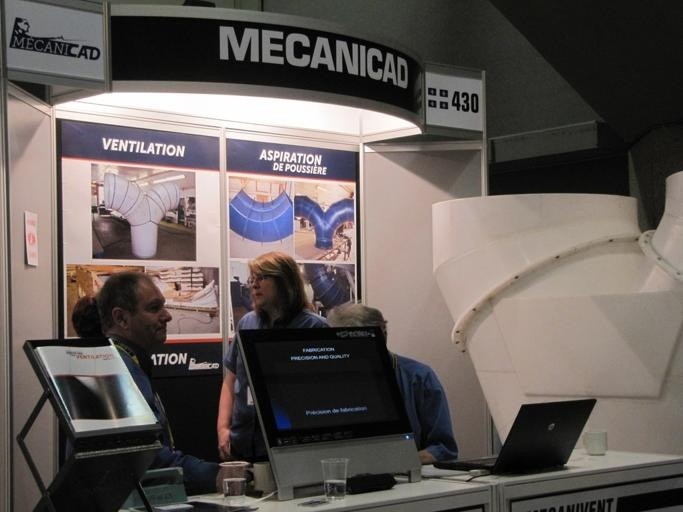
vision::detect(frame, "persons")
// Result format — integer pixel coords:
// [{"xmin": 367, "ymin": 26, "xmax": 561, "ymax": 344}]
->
[
  {"xmin": 216, "ymin": 248, "xmax": 326, "ymax": 464},
  {"xmin": 96, "ymin": 270, "xmax": 250, "ymax": 493},
  {"xmin": 326, "ymin": 299, "xmax": 459, "ymax": 464},
  {"xmin": 59, "ymin": 294, "xmax": 107, "ymax": 494}
]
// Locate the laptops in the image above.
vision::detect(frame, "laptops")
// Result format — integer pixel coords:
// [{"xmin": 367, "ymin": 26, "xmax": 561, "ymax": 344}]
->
[{"xmin": 433, "ymin": 398, "xmax": 597, "ymax": 474}]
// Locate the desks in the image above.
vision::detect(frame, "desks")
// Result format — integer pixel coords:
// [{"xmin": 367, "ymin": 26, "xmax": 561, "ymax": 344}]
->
[
  {"xmin": 420, "ymin": 446, "xmax": 681, "ymax": 512},
  {"xmin": 125, "ymin": 476, "xmax": 496, "ymax": 512}
]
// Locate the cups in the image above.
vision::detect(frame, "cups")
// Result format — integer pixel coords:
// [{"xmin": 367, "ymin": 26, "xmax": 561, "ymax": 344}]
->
[
  {"xmin": 252, "ymin": 460, "xmax": 275, "ymax": 494},
  {"xmin": 583, "ymin": 430, "xmax": 608, "ymax": 455},
  {"xmin": 218, "ymin": 460, "xmax": 248, "ymax": 506},
  {"xmin": 319, "ymin": 455, "xmax": 349, "ymax": 501}
]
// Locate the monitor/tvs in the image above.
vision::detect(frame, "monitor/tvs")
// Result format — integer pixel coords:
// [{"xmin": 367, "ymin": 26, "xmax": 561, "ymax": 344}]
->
[{"xmin": 235, "ymin": 327, "xmax": 422, "ymax": 503}]
